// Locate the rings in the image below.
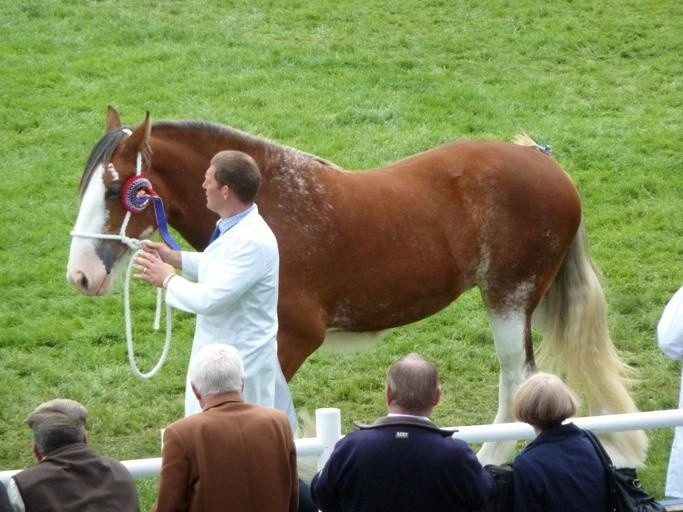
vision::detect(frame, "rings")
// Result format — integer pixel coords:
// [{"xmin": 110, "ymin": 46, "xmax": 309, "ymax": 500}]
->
[{"xmin": 143, "ymin": 266, "xmax": 147, "ymax": 273}]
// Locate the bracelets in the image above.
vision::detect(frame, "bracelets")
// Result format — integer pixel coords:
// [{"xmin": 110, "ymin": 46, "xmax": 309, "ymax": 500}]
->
[{"xmin": 163, "ymin": 271, "xmax": 176, "ymax": 288}]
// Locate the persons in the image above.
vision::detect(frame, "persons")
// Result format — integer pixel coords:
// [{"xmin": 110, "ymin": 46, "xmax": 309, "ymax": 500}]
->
[
  {"xmin": 0, "ymin": 397, "xmax": 139, "ymax": 512},
  {"xmin": 510, "ymin": 373, "xmax": 617, "ymax": 512},
  {"xmin": 311, "ymin": 352, "xmax": 497, "ymax": 512},
  {"xmin": 152, "ymin": 344, "xmax": 301, "ymax": 512},
  {"xmin": 132, "ymin": 150, "xmax": 302, "ymax": 442},
  {"xmin": 657, "ymin": 286, "xmax": 683, "ymax": 498}
]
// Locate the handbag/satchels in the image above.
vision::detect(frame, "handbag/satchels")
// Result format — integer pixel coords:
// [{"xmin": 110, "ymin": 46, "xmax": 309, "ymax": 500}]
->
[
  {"xmin": 483, "ymin": 462, "xmax": 515, "ymax": 512},
  {"xmin": 582, "ymin": 428, "xmax": 668, "ymax": 512}
]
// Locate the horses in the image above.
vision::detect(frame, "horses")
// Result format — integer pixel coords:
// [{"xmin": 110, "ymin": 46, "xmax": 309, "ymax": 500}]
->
[{"xmin": 65, "ymin": 104, "xmax": 653, "ymax": 472}]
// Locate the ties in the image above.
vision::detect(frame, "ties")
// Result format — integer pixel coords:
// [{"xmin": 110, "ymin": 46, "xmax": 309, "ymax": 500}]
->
[{"xmin": 207, "ymin": 224, "xmax": 220, "ymax": 246}]
